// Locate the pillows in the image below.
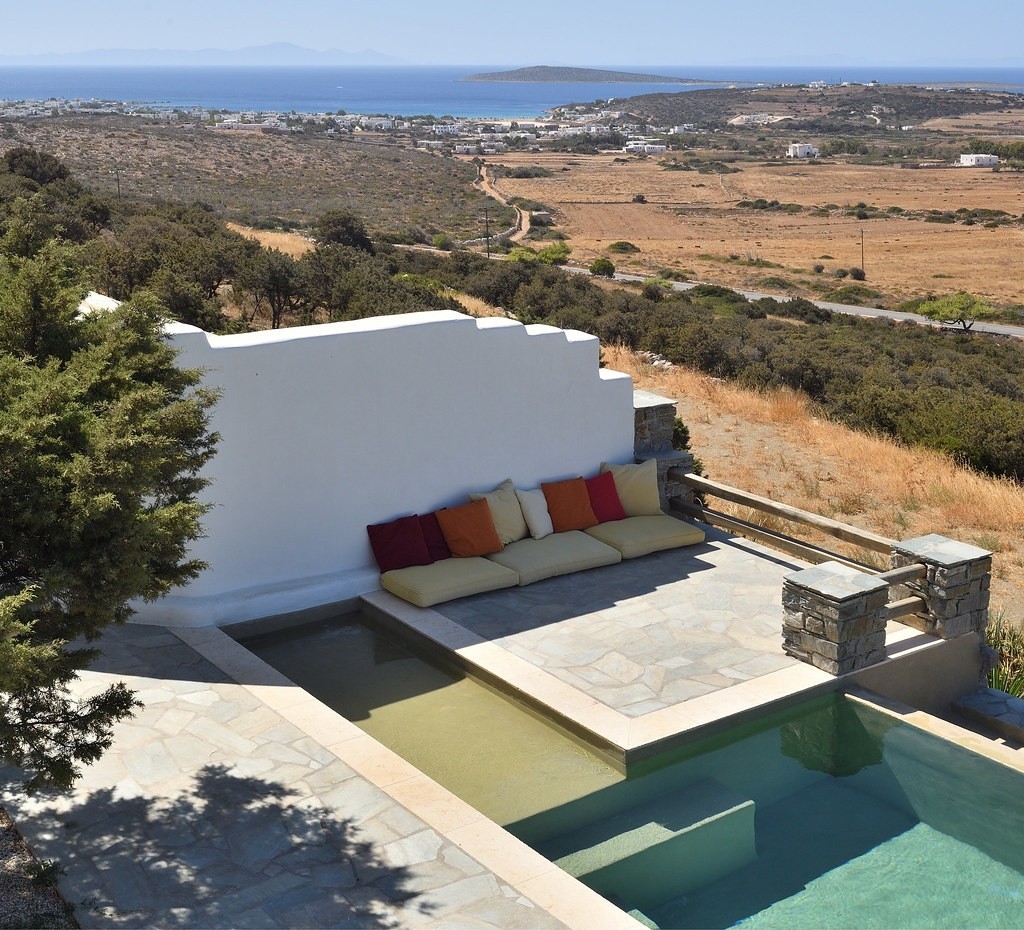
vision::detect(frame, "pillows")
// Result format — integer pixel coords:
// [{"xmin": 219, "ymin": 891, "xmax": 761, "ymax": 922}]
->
[
  {"xmin": 541, "ymin": 476, "xmax": 599, "ymax": 533},
  {"xmin": 468, "ymin": 479, "xmax": 530, "ymax": 547},
  {"xmin": 434, "ymin": 497, "xmax": 503, "ymax": 557},
  {"xmin": 584, "ymin": 471, "xmax": 626, "ymax": 524},
  {"xmin": 367, "ymin": 513, "xmax": 431, "ymax": 575},
  {"xmin": 419, "ymin": 507, "xmax": 452, "ymax": 562},
  {"xmin": 515, "ymin": 489, "xmax": 554, "ymax": 540},
  {"xmin": 600, "ymin": 457, "xmax": 665, "ymax": 517}
]
